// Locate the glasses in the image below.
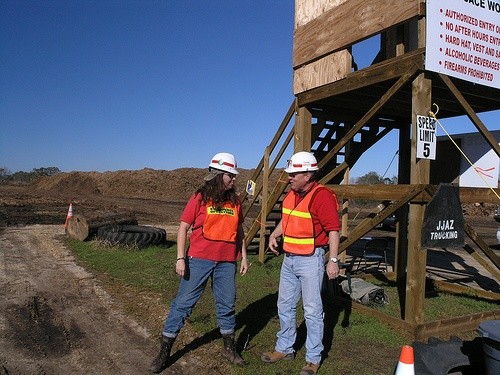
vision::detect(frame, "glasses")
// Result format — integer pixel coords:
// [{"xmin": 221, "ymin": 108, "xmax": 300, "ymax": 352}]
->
[
  {"xmin": 288, "ymin": 171, "xmax": 311, "ymax": 178},
  {"xmin": 223, "ymin": 173, "xmax": 236, "ymax": 179}
]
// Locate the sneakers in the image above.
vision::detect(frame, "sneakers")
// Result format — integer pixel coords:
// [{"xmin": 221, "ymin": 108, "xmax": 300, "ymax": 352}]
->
[
  {"xmin": 300, "ymin": 360, "xmax": 319, "ymax": 375},
  {"xmin": 261, "ymin": 350, "xmax": 296, "ymax": 362}
]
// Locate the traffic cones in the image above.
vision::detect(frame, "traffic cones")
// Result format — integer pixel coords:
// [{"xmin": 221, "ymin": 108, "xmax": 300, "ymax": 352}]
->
[
  {"xmin": 64, "ymin": 203, "xmax": 73, "ymax": 234},
  {"xmin": 394, "ymin": 345, "xmax": 416, "ymax": 375}
]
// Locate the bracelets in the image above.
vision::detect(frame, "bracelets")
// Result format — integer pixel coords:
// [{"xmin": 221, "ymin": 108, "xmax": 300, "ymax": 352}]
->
[{"xmin": 177, "ymin": 257, "xmax": 185, "ymax": 261}]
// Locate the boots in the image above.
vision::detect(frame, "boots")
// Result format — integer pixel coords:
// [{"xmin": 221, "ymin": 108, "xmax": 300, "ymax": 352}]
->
[
  {"xmin": 150, "ymin": 334, "xmax": 177, "ymax": 372},
  {"xmin": 222, "ymin": 333, "xmax": 245, "ymax": 366}
]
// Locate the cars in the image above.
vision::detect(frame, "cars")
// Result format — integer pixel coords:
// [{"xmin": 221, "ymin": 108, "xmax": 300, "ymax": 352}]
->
[{"xmin": 374, "ymin": 208, "xmax": 397, "ymax": 230}]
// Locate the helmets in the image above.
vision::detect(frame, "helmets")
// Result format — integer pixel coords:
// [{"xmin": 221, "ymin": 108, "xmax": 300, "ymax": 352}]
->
[
  {"xmin": 284, "ymin": 151, "xmax": 319, "ymax": 173},
  {"xmin": 209, "ymin": 153, "xmax": 239, "ymax": 174}
]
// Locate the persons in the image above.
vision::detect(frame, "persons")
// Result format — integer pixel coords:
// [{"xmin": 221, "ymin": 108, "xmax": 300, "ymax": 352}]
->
[
  {"xmin": 147, "ymin": 152, "xmax": 248, "ymax": 373},
  {"xmin": 261, "ymin": 151, "xmax": 342, "ymax": 375}
]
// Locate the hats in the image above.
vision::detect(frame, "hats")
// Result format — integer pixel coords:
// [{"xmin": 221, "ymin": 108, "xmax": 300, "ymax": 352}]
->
[{"xmin": 203, "ymin": 168, "xmax": 236, "ymax": 181}]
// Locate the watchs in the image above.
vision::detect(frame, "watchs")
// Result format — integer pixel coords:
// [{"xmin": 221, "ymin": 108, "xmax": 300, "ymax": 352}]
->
[{"xmin": 328, "ymin": 257, "xmax": 338, "ymax": 263}]
